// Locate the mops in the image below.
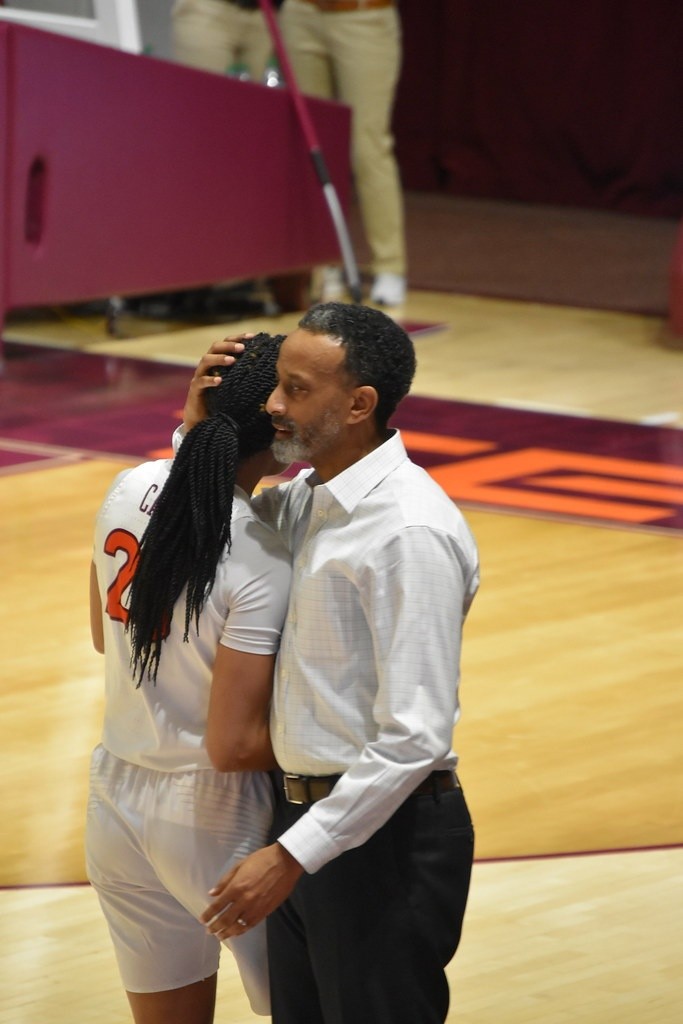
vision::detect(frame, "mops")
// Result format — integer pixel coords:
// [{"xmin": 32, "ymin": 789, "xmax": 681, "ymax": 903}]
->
[{"xmin": 257, "ymin": 0, "xmax": 449, "ymax": 341}]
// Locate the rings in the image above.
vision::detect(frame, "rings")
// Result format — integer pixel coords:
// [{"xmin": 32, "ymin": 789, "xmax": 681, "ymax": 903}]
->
[{"xmin": 237, "ymin": 919, "xmax": 248, "ymax": 928}]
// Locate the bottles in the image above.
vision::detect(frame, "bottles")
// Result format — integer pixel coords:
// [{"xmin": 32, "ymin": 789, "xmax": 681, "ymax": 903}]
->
[
  {"xmin": 222, "ymin": 40, "xmax": 253, "ymax": 83},
  {"xmin": 263, "ymin": 45, "xmax": 292, "ymax": 87}
]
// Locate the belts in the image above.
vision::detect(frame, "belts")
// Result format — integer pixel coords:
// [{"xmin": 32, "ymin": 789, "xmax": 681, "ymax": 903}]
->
[{"xmin": 283, "ymin": 769, "xmax": 461, "ymax": 805}]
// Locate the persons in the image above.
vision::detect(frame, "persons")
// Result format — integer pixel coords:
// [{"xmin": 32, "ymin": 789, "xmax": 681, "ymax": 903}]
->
[
  {"xmin": 90, "ymin": 331, "xmax": 291, "ymax": 1024},
  {"xmin": 172, "ymin": 299, "xmax": 475, "ymax": 1024},
  {"xmin": 173, "ymin": 1, "xmax": 414, "ymax": 306}
]
